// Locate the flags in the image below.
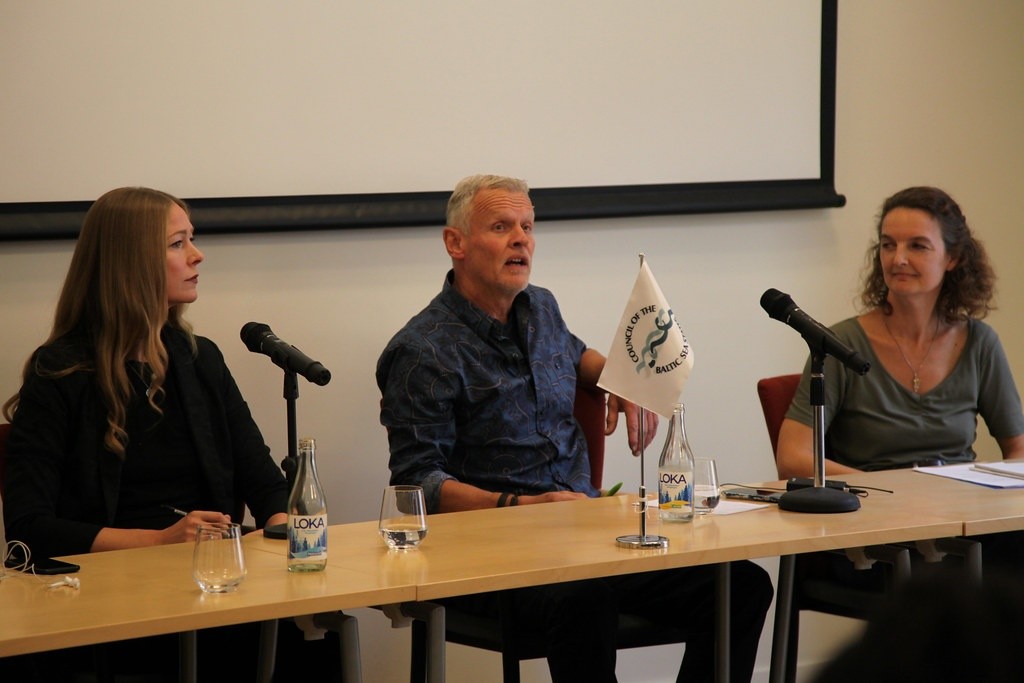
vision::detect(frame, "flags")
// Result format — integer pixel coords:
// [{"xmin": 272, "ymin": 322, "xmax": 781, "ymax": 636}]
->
[{"xmin": 595, "ymin": 250, "xmax": 695, "ymax": 421}]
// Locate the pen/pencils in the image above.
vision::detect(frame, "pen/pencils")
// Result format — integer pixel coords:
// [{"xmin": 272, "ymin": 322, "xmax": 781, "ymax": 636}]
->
[
  {"xmin": 607, "ymin": 482, "xmax": 622, "ymax": 496},
  {"xmin": 159, "ymin": 504, "xmax": 188, "ymax": 516}
]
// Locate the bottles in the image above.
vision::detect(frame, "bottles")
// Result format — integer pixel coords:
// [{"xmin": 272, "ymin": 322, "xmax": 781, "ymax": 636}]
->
[
  {"xmin": 286, "ymin": 438, "xmax": 329, "ymax": 573},
  {"xmin": 658, "ymin": 403, "xmax": 694, "ymax": 522}
]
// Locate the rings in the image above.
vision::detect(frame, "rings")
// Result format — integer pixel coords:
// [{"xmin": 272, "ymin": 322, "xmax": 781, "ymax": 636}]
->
[{"xmin": 210, "ymin": 534, "xmax": 214, "ymax": 540}]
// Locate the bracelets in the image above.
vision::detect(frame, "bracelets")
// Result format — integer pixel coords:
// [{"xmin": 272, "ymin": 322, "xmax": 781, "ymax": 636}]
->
[{"xmin": 494, "ymin": 489, "xmax": 519, "ymax": 510}]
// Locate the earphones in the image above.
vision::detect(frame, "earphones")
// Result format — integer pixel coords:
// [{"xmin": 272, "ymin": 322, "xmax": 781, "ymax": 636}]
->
[
  {"xmin": 69, "ymin": 579, "xmax": 80, "ymax": 590},
  {"xmin": 47, "ymin": 577, "xmax": 73, "ymax": 588}
]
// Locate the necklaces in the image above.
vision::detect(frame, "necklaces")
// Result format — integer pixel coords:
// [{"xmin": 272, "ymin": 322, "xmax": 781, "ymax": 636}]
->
[{"xmin": 885, "ymin": 307, "xmax": 945, "ymax": 391}]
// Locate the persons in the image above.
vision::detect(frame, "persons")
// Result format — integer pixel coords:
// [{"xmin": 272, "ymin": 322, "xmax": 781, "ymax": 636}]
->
[
  {"xmin": 775, "ymin": 187, "xmax": 1024, "ymax": 619},
  {"xmin": 378, "ymin": 171, "xmax": 773, "ymax": 683},
  {"xmin": 1, "ymin": 185, "xmax": 330, "ymax": 555}
]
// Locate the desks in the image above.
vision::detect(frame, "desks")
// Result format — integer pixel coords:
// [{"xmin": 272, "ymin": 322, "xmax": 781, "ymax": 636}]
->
[{"xmin": 0, "ymin": 461, "xmax": 1024, "ymax": 683}]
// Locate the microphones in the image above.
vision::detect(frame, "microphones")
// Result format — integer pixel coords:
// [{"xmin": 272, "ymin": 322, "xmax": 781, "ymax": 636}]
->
[
  {"xmin": 759, "ymin": 288, "xmax": 871, "ymax": 376},
  {"xmin": 240, "ymin": 322, "xmax": 331, "ymax": 386}
]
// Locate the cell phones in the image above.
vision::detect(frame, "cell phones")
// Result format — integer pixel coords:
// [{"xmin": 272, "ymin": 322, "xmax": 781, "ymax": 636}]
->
[
  {"xmin": 722, "ymin": 488, "xmax": 784, "ymax": 502},
  {"xmin": 5, "ymin": 557, "xmax": 80, "ymax": 575}
]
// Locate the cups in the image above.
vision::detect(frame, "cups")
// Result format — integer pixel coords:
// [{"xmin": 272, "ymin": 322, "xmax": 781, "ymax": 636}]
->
[
  {"xmin": 691, "ymin": 457, "xmax": 721, "ymax": 515},
  {"xmin": 191, "ymin": 522, "xmax": 246, "ymax": 594},
  {"xmin": 378, "ymin": 485, "xmax": 429, "ymax": 550}
]
